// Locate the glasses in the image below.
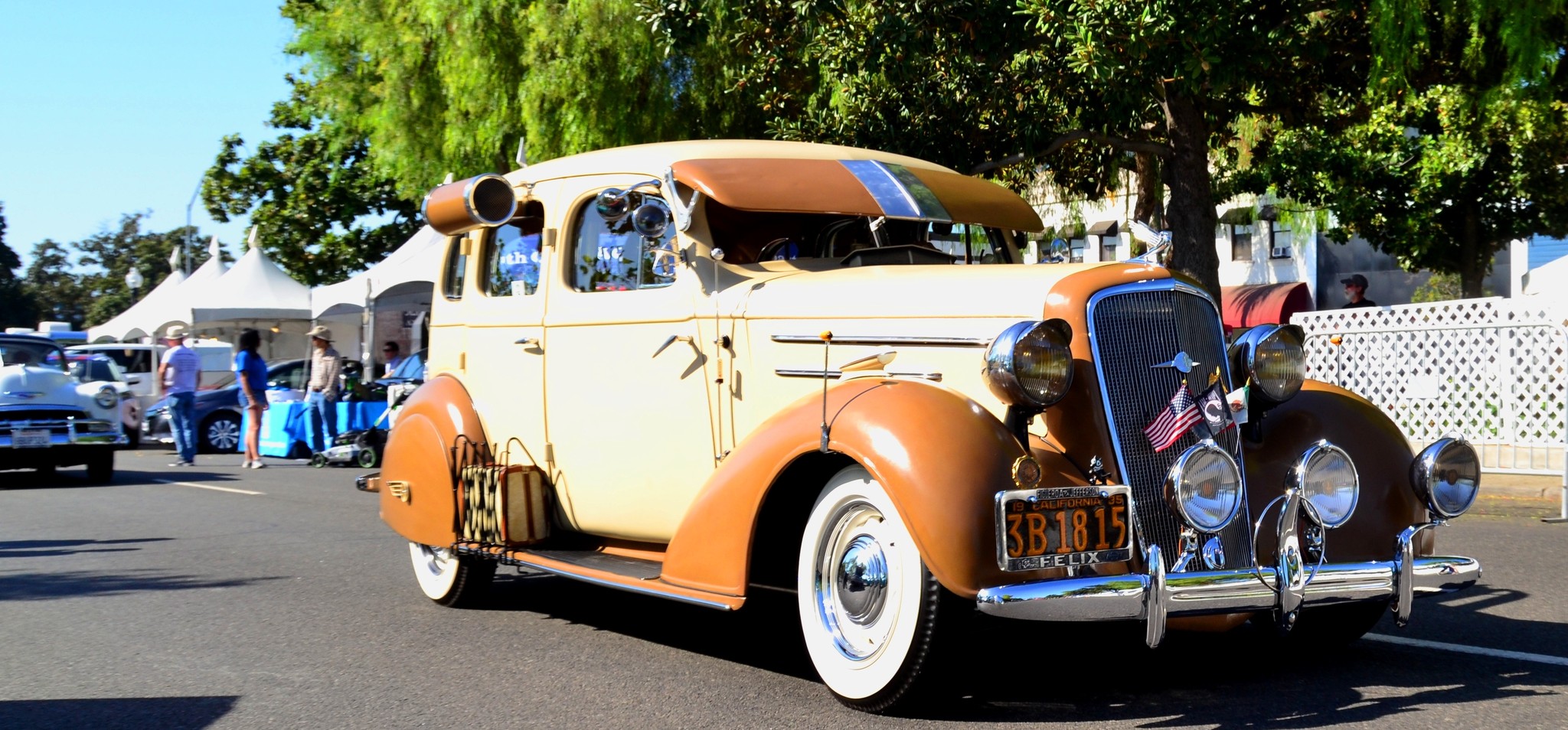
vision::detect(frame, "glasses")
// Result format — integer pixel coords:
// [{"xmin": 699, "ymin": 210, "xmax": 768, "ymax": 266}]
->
[{"xmin": 382, "ymin": 348, "xmax": 394, "ymax": 352}]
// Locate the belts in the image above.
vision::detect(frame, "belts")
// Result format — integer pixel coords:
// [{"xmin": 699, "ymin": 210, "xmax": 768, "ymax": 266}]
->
[{"xmin": 312, "ymin": 387, "xmax": 325, "ymax": 393}]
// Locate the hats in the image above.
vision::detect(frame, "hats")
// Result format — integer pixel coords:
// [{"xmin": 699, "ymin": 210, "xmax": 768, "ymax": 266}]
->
[
  {"xmin": 305, "ymin": 326, "xmax": 336, "ymax": 343},
  {"xmin": 163, "ymin": 325, "xmax": 189, "ymax": 339},
  {"xmin": 1340, "ymin": 273, "xmax": 1369, "ymax": 290}
]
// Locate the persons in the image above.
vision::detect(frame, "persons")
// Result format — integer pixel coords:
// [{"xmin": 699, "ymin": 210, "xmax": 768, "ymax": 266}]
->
[
  {"xmin": 1342, "ymin": 274, "xmax": 1376, "ymax": 309},
  {"xmin": 380, "ymin": 341, "xmax": 403, "ymax": 379},
  {"xmin": 157, "ymin": 325, "xmax": 201, "ymax": 467},
  {"xmin": 307, "ymin": 326, "xmax": 342, "ymax": 466},
  {"xmin": 233, "ymin": 327, "xmax": 269, "ymax": 469}
]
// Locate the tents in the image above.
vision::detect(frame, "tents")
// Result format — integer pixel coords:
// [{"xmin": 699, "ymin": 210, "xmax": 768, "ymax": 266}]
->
[{"xmin": 92, "ymin": 172, "xmax": 462, "ymax": 384}]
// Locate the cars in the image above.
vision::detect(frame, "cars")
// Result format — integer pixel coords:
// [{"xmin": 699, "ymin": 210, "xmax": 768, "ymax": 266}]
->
[
  {"xmin": 0, "ymin": 333, "xmax": 129, "ymax": 483},
  {"xmin": 351, "ymin": 140, "xmax": 1485, "ymax": 714},
  {"xmin": 140, "ymin": 358, "xmax": 364, "ymax": 457},
  {"xmin": 65, "ymin": 353, "xmax": 144, "ymax": 453}
]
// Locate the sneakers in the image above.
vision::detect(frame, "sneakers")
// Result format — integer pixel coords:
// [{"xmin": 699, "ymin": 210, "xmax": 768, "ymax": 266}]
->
[
  {"xmin": 251, "ymin": 459, "xmax": 266, "ymax": 469},
  {"xmin": 168, "ymin": 458, "xmax": 194, "ymax": 467},
  {"xmin": 242, "ymin": 460, "xmax": 252, "ymax": 468}
]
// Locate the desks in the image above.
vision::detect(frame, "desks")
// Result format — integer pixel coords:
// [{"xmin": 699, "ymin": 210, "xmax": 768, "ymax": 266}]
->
[{"xmin": 236, "ymin": 401, "xmax": 388, "ymax": 459}]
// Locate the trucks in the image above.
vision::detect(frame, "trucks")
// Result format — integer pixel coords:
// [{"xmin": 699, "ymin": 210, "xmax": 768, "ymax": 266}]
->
[{"xmin": 65, "ymin": 336, "xmax": 233, "ymax": 432}]
